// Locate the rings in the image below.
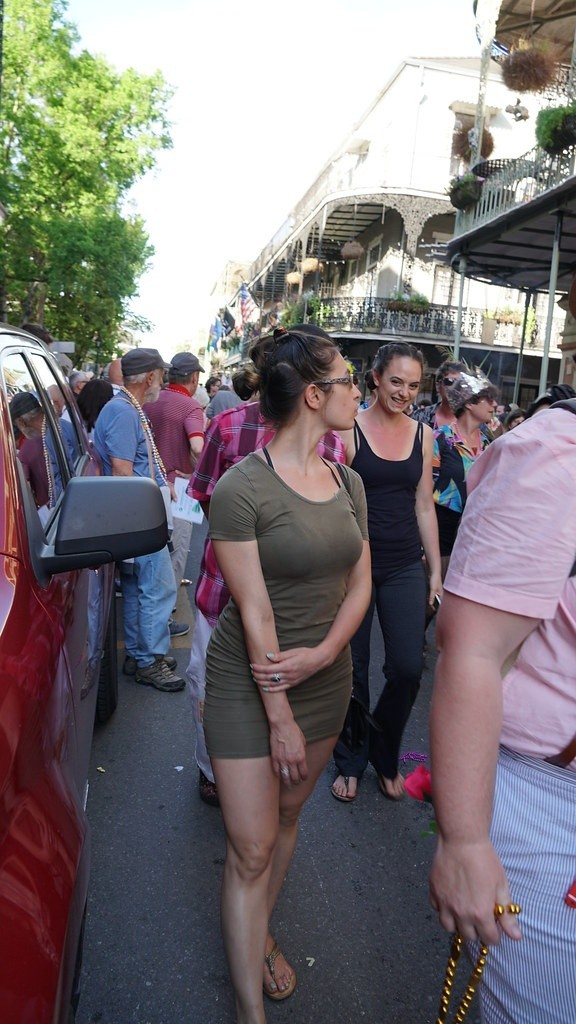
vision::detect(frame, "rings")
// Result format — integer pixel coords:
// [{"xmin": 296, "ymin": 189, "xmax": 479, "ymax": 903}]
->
[
  {"xmin": 281, "ymin": 767, "xmax": 289, "ymax": 775},
  {"xmin": 272, "ymin": 673, "xmax": 281, "ymax": 682}
]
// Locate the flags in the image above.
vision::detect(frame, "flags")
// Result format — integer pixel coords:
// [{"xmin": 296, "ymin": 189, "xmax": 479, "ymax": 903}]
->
[{"xmin": 208, "ymin": 281, "xmax": 256, "ymax": 353}]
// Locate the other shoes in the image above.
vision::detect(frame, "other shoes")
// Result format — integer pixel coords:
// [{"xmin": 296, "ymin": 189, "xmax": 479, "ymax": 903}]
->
[{"xmin": 199, "ymin": 768, "xmax": 219, "ymax": 806}]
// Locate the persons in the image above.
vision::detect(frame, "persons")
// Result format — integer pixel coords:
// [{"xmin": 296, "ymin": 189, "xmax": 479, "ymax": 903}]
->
[
  {"xmin": 359, "ymin": 363, "xmax": 576, "ymax": 665},
  {"xmin": 187, "ymin": 324, "xmax": 347, "ymax": 802},
  {"xmin": 11, "ymin": 392, "xmax": 82, "ymax": 509},
  {"xmin": 203, "ymin": 330, "xmax": 372, "ymax": 1024},
  {"xmin": 93, "ymin": 348, "xmax": 186, "ymax": 691},
  {"xmin": 144, "ymin": 352, "xmax": 250, "ymax": 635},
  {"xmin": 276, "ymin": 302, "xmax": 285, "ymax": 319},
  {"xmin": 428, "ymin": 398, "xmax": 576, "ymax": 1024},
  {"xmin": 331, "ymin": 344, "xmax": 443, "ymax": 802},
  {"xmin": 21, "ymin": 322, "xmax": 124, "ymax": 441},
  {"xmin": 13, "ymin": 428, "xmax": 53, "ymax": 520},
  {"xmin": 47, "ymin": 385, "xmax": 71, "ymax": 422}
]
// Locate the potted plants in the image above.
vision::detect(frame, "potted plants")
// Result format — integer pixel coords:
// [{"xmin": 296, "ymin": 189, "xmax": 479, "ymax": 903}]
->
[{"xmin": 535, "ymin": 98, "xmax": 576, "ymax": 155}]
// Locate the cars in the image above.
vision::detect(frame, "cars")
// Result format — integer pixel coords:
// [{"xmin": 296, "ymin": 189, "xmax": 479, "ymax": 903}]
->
[{"xmin": 0, "ymin": 320, "xmax": 171, "ymax": 1024}]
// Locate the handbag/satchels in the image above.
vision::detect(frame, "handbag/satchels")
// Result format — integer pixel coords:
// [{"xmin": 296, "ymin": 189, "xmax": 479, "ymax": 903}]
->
[{"xmin": 340, "ymin": 692, "xmax": 377, "ymax": 752}]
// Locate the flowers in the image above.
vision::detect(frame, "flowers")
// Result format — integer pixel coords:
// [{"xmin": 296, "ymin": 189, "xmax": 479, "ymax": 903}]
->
[
  {"xmin": 344, "ymin": 355, "xmax": 357, "ymax": 374},
  {"xmin": 443, "ymin": 168, "xmax": 478, "ymax": 197}
]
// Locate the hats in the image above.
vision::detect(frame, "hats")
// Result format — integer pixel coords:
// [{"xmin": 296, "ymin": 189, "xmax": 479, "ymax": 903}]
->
[
  {"xmin": 10, "ymin": 392, "xmax": 39, "ymax": 419},
  {"xmin": 170, "ymin": 352, "xmax": 205, "ymax": 375},
  {"xmin": 121, "ymin": 348, "xmax": 173, "ymax": 376},
  {"xmin": 445, "ymin": 364, "xmax": 492, "ymax": 414}
]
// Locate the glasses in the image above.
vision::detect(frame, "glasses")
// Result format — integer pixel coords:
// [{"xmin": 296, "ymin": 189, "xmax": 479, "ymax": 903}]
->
[
  {"xmin": 476, "ymin": 394, "xmax": 494, "ymax": 403},
  {"xmin": 213, "ymin": 385, "xmax": 220, "ymax": 387},
  {"xmin": 309, "ymin": 372, "xmax": 358, "ymax": 389},
  {"xmin": 441, "ymin": 378, "xmax": 455, "ymax": 386}
]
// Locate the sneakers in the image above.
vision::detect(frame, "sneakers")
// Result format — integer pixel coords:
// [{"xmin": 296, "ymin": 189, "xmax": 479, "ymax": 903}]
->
[
  {"xmin": 124, "ymin": 655, "xmax": 176, "ymax": 675},
  {"xmin": 136, "ymin": 655, "xmax": 186, "ymax": 692},
  {"xmin": 169, "ymin": 621, "xmax": 189, "ymax": 637}
]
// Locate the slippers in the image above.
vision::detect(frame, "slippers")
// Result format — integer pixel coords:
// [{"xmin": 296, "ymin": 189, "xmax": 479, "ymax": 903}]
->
[
  {"xmin": 263, "ymin": 943, "xmax": 296, "ymax": 1000},
  {"xmin": 331, "ymin": 774, "xmax": 358, "ymax": 801},
  {"xmin": 377, "ymin": 773, "xmax": 405, "ymax": 798}
]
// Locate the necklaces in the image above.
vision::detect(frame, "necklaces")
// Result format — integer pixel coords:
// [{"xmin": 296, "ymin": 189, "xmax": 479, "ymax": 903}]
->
[
  {"xmin": 436, "ymin": 904, "xmax": 521, "ymax": 1024},
  {"xmin": 120, "ymin": 385, "xmax": 169, "ymax": 486},
  {"xmin": 42, "ymin": 416, "xmax": 62, "ymax": 509}
]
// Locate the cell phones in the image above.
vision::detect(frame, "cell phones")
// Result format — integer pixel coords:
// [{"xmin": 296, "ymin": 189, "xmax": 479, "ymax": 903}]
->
[{"xmin": 434, "ymin": 594, "xmax": 441, "ymax": 610}]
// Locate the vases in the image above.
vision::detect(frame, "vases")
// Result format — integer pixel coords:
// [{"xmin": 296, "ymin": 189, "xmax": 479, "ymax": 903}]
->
[{"xmin": 451, "ymin": 181, "xmax": 482, "ymax": 210}]
